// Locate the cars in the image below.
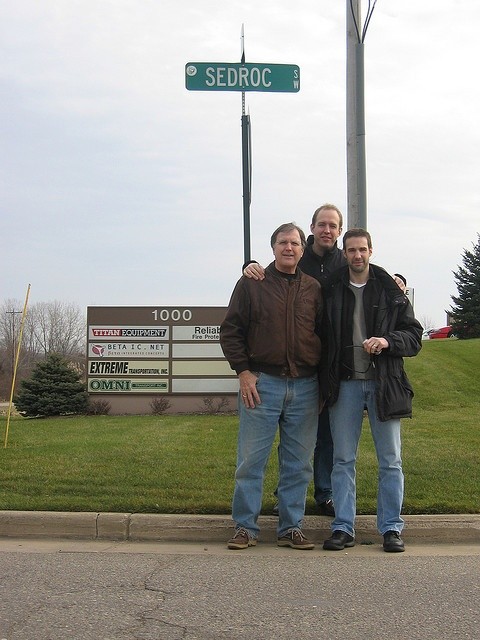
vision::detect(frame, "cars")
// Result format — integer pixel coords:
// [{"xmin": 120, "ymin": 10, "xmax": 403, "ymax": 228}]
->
[{"xmin": 421, "ymin": 325, "xmax": 468, "ymax": 338}]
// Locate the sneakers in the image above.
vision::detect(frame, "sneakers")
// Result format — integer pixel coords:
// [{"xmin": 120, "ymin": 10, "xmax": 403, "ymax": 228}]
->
[
  {"xmin": 226, "ymin": 527, "xmax": 258, "ymax": 549},
  {"xmin": 319, "ymin": 498, "xmax": 335, "ymax": 517},
  {"xmin": 322, "ymin": 530, "xmax": 355, "ymax": 550},
  {"xmin": 276, "ymin": 527, "xmax": 315, "ymax": 549},
  {"xmin": 272, "ymin": 498, "xmax": 279, "ymax": 516},
  {"xmin": 383, "ymin": 530, "xmax": 406, "ymax": 552}
]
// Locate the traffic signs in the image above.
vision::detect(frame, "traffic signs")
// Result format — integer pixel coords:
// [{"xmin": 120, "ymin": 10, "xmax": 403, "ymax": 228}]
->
[{"xmin": 185, "ymin": 61, "xmax": 301, "ymax": 94}]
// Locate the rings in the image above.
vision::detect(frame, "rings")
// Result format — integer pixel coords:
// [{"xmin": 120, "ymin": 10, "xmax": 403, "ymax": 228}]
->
[
  {"xmin": 242, "ymin": 394, "xmax": 247, "ymax": 398},
  {"xmin": 373, "ymin": 345, "xmax": 377, "ymax": 347}
]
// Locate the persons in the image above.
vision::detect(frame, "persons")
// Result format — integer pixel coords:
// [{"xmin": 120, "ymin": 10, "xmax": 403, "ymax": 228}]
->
[
  {"xmin": 241, "ymin": 203, "xmax": 406, "ymax": 519},
  {"xmin": 218, "ymin": 223, "xmax": 323, "ymax": 549},
  {"xmin": 314, "ymin": 227, "xmax": 424, "ymax": 552}
]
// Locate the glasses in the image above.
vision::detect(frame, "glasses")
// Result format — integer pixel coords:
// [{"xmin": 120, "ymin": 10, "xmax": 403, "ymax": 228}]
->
[{"xmin": 340, "ymin": 345, "xmax": 374, "ymax": 374}]
